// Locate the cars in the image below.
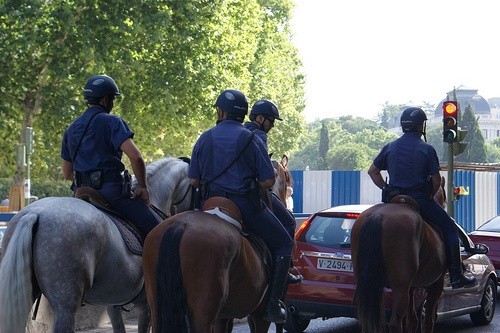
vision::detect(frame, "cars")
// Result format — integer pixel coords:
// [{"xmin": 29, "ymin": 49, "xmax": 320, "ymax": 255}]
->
[
  {"xmin": 460, "ymin": 215, "xmax": 499, "ymax": 285},
  {"xmin": 280, "ymin": 203, "xmax": 499, "ymax": 332}
]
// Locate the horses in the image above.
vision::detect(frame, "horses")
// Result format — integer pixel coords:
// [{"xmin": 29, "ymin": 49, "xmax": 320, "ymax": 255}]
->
[
  {"xmin": 142, "ymin": 152, "xmax": 294, "ymax": 333},
  {"xmin": 0, "ymin": 156, "xmax": 194, "ymax": 333},
  {"xmin": 349, "ymin": 175, "xmax": 454, "ymax": 333}
]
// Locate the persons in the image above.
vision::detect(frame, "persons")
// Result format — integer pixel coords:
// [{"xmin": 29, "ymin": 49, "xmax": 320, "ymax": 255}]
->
[
  {"xmin": 244, "ymin": 97, "xmax": 303, "ymax": 287},
  {"xmin": 60, "ymin": 73, "xmax": 162, "ymax": 245},
  {"xmin": 367, "ymin": 107, "xmax": 473, "ymax": 290},
  {"xmin": 186, "ymin": 90, "xmax": 295, "ymax": 326}
]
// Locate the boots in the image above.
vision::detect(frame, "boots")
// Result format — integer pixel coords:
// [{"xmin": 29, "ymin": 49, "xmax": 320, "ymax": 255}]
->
[
  {"xmin": 445, "ymin": 243, "xmax": 476, "ymax": 288},
  {"xmin": 264, "ymin": 253, "xmax": 304, "ymax": 322},
  {"xmin": 288, "ymin": 232, "xmax": 303, "ymax": 284}
]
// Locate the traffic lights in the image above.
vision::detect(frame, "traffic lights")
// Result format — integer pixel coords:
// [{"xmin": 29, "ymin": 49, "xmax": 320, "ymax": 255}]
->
[
  {"xmin": 452, "ymin": 186, "xmax": 460, "ymax": 201},
  {"xmin": 453, "ymin": 126, "xmax": 469, "ymax": 155},
  {"xmin": 443, "ymin": 101, "xmax": 457, "ymax": 142}
]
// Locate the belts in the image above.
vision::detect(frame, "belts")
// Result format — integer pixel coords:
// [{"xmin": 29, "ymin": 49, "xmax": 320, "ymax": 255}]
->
[{"xmin": 210, "ymin": 191, "xmax": 246, "ymax": 198}]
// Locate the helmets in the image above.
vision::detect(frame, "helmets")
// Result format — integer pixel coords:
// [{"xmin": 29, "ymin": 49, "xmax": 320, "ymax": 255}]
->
[
  {"xmin": 251, "ymin": 100, "xmax": 283, "ymax": 120},
  {"xmin": 213, "ymin": 90, "xmax": 248, "ymax": 116},
  {"xmin": 83, "ymin": 74, "xmax": 121, "ymax": 102},
  {"xmin": 400, "ymin": 107, "xmax": 428, "ymax": 141}
]
